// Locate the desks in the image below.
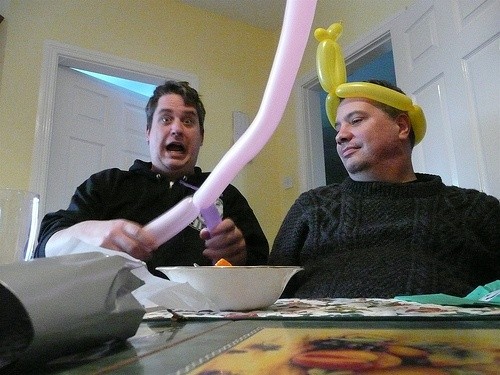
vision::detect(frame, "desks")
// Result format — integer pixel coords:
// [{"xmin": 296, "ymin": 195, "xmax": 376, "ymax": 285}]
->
[{"xmin": 0, "ymin": 298, "xmax": 500, "ymax": 375}]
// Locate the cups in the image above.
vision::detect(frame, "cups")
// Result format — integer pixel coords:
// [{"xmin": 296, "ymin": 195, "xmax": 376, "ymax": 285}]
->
[{"xmin": 0, "ymin": 188, "xmax": 40, "ymax": 264}]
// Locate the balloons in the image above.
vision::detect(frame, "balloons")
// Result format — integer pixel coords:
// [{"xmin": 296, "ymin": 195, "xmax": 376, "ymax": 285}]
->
[
  {"xmin": 141, "ymin": 0, "xmax": 319, "ymax": 249},
  {"xmin": 314, "ymin": 22, "xmax": 427, "ymax": 146}
]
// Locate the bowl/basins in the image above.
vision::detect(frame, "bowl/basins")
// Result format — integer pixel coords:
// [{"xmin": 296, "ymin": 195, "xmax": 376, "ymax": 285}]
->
[{"xmin": 156, "ymin": 266, "xmax": 305, "ymax": 311}]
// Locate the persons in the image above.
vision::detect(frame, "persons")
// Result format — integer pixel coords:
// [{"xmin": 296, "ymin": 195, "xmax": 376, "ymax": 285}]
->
[
  {"xmin": 268, "ymin": 80, "xmax": 500, "ymax": 298},
  {"xmin": 24, "ymin": 79, "xmax": 270, "ymax": 281}
]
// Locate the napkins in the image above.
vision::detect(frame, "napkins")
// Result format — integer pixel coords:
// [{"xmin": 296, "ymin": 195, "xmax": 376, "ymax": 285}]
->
[{"xmin": 64, "ymin": 238, "xmax": 218, "ymax": 315}]
